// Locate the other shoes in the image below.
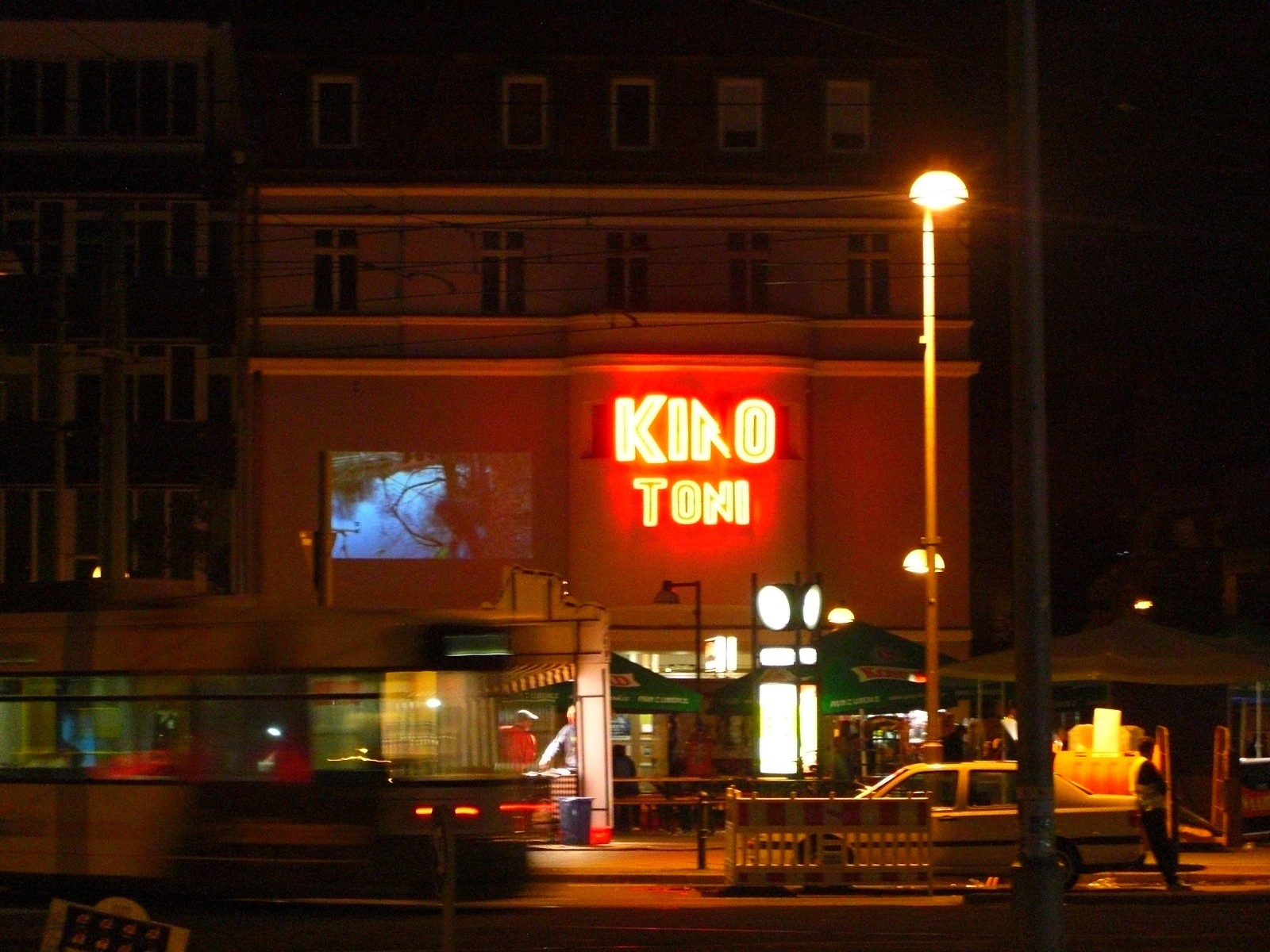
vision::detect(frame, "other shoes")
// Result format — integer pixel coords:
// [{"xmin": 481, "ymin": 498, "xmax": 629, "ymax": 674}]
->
[{"xmin": 1167, "ymin": 885, "xmax": 1191, "ymax": 892}]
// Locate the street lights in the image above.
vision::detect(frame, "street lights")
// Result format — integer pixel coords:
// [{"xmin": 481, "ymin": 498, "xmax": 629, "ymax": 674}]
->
[
  {"xmin": 652, "ymin": 578, "xmax": 714, "ymax": 836},
  {"xmin": 910, "ymin": 169, "xmax": 971, "ymax": 796},
  {"xmin": 754, "ymin": 568, "xmax": 824, "ymax": 777}
]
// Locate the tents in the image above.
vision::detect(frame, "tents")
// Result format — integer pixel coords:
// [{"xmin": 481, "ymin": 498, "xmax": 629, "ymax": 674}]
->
[{"xmin": 937, "ymin": 618, "xmax": 1270, "ymax": 803}]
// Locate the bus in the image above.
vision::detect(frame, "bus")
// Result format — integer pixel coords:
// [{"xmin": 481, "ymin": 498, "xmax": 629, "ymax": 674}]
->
[{"xmin": 0, "ymin": 576, "xmax": 585, "ymax": 914}]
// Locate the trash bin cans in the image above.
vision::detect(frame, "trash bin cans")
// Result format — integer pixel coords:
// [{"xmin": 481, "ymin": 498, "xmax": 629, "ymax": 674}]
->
[{"xmin": 560, "ymin": 797, "xmax": 592, "ymax": 844}]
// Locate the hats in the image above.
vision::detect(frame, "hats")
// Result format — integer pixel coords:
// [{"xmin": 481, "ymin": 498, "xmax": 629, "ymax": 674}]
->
[{"xmin": 518, "ymin": 709, "xmax": 539, "ymax": 719}]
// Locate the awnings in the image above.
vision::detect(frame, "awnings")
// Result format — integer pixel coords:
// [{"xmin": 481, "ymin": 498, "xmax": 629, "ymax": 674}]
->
[{"xmin": 478, "ymin": 663, "xmax": 574, "ymax": 697}]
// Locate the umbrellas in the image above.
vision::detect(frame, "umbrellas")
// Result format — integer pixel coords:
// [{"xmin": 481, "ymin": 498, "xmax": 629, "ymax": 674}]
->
[
  {"xmin": 968, "ymin": 679, "xmax": 1109, "ymax": 708},
  {"xmin": 710, "ymin": 619, "xmax": 984, "ymax": 778},
  {"xmin": 497, "ymin": 653, "xmax": 702, "ymax": 712}
]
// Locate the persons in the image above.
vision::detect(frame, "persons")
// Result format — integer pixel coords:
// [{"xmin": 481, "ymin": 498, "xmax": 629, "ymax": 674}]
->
[
  {"xmin": 613, "ymin": 745, "xmax": 641, "ymax": 832},
  {"xmin": 498, "ymin": 708, "xmax": 539, "ymax": 763},
  {"xmin": 983, "ymin": 739, "xmax": 1003, "ymax": 761},
  {"xmin": 1129, "ymin": 734, "xmax": 1193, "ymax": 890},
  {"xmin": 941, "ymin": 713, "xmax": 986, "ymax": 762},
  {"xmin": 539, "ymin": 705, "xmax": 577, "ymax": 768},
  {"xmin": 816, "ymin": 734, "xmax": 860, "ymax": 779},
  {"xmin": 686, "ymin": 728, "xmax": 715, "ymax": 775},
  {"xmin": 654, "ymin": 757, "xmax": 690, "ymax": 836}
]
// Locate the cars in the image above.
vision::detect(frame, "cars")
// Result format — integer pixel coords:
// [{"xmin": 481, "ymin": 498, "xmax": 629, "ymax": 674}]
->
[{"xmin": 743, "ymin": 757, "xmax": 1147, "ymax": 893}]
